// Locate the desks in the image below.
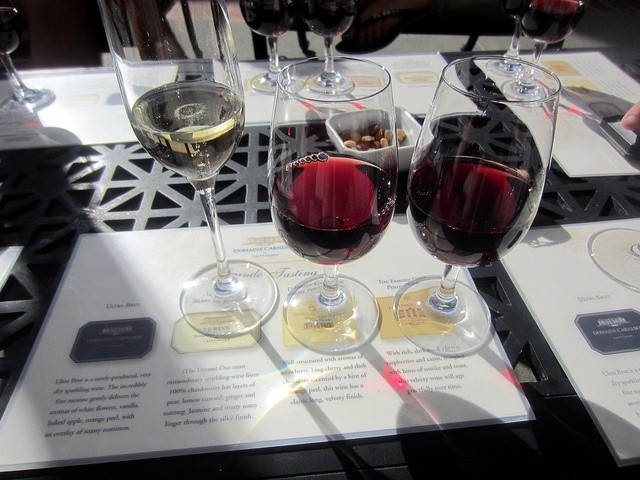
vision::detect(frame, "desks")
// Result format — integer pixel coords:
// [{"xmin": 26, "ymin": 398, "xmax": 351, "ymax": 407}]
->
[{"xmin": 0, "ymin": 49, "xmax": 640, "ymax": 479}]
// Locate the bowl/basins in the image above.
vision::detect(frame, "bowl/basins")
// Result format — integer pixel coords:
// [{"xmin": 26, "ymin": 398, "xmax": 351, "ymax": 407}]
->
[{"xmin": 324, "ymin": 107, "xmax": 435, "ymax": 172}]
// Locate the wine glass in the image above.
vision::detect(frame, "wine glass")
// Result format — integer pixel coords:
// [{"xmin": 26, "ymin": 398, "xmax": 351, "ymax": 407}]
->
[
  {"xmin": 588, "ymin": 228, "xmax": 640, "ymax": 293},
  {"xmin": 486, "ymin": 0, "xmax": 534, "ymax": 78},
  {"xmin": 239, "ymin": 0, "xmax": 297, "ymax": 93},
  {"xmin": 266, "ymin": 56, "xmax": 400, "ymax": 352},
  {"xmin": 298, "ymin": 1, "xmax": 356, "ymax": 97},
  {"xmin": 0, "ymin": 0, "xmax": 57, "ymax": 116},
  {"xmin": 98, "ymin": 0, "xmax": 280, "ymax": 339},
  {"xmin": 501, "ymin": 0, "xmax": 585, "ymax": 108},
  {"xmin": 392, "ymin": 54, "xmax": 562, "ymax": 359}
]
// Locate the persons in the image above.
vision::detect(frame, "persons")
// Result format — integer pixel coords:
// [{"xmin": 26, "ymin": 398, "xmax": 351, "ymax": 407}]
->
[{"xmin": 620, "ymin": 101, "xmax": 639, "ymax": 134}]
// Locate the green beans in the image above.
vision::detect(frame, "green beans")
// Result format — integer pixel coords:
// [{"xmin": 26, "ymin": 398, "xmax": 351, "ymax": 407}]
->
[{"xmin": 339, "ymin": 125, "xmax": 404, "ymax": 150}]
